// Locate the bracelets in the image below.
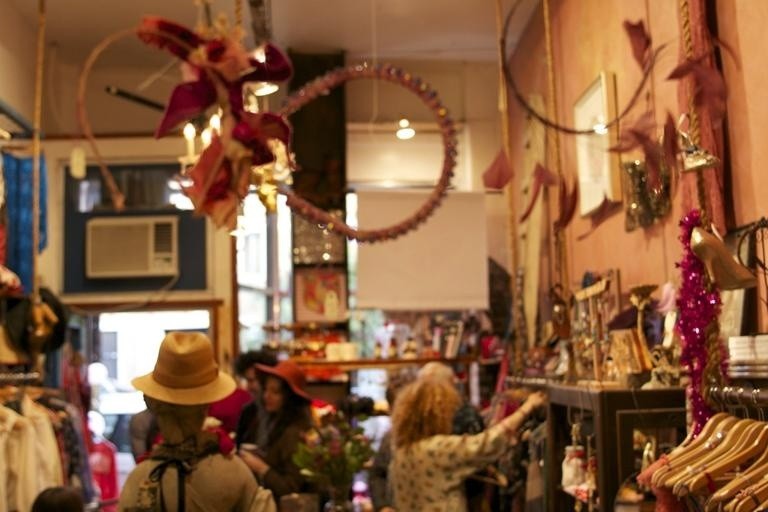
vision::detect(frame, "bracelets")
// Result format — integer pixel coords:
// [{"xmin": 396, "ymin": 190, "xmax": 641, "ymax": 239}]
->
[{"xmin": 520, "ymin": 409, "xmax": 526, "ymax": 417}]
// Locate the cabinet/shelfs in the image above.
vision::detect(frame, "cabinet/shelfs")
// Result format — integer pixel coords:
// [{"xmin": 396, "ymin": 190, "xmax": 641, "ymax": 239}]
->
[{"xmin": 503, "ymin": 379, "xmax": 685, "ymax": 512}]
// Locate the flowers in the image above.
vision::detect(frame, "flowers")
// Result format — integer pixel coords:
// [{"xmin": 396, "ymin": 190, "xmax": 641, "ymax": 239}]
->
[{"xmin": 296, "ymin": 397, "xmax": 375, "ymax": 487}]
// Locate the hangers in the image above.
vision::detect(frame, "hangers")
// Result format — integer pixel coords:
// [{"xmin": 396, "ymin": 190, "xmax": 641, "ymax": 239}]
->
[{"xmin": 652, "ymin": 382, "xmax": 767, "ymax": 511}]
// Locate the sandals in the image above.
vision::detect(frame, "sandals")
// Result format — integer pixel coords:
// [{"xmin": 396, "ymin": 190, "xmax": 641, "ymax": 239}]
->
[{"xmin": 658, "ymin": 114, "xmax": 720, "ymax": 171}]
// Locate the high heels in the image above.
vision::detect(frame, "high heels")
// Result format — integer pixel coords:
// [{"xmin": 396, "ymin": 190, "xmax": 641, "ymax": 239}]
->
[{"xmin": 691, "ymin": 228, "xmax": 758, "ymax": 290}]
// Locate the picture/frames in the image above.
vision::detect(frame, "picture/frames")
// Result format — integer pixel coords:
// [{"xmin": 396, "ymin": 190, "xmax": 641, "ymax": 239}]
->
[
  {"xmin": 569, "ymin": 71, "xmax": 621, "ymax": 219},
  {"xmin": 290, "ymin": 207, "xmax": 348, "ymax": 388}
]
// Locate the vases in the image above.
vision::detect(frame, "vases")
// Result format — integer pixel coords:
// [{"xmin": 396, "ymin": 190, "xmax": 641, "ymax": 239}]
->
[{"xmin": 326, "ymin": 488, "xmax": 357, "ymax": 511}]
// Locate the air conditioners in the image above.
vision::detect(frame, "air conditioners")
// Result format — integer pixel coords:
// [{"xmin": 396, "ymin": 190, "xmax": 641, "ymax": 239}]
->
[{"xmin": 82, "ymin": 214, "xmax": 178, "ymax": 283}]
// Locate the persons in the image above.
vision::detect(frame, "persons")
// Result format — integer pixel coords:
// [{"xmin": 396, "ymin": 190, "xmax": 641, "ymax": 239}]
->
[
  {"xmin": 116, "ymin": 330, "xmax": 277, "ymax": 510},
  {"xmin": 234, "ymin": 351, "xmax": 279, "ymax": 395},
  {"xmin": 386, "ymin": 377, "xmax": 549, "ymax": 511},
  {"xmin": 33, "ymin": 486, "xmax": 85, "ymax": 511},
  {"xmin": 207, "ymin": 390, "xmax": 252, "ymax": 436},
  {"xmin": 130, "ymin": 393, "xmax": 161, "ymax": 465},
  {"xmin": 419, "ymin": 362, "xmax": 488, "ymax": 511},
  {"xmin": 239, "ymin": 361, "xmax": 314, "ymax": 505},
  {"xmin": 369, "ymin": 378, "xmax": 415, "ymax": 511}
]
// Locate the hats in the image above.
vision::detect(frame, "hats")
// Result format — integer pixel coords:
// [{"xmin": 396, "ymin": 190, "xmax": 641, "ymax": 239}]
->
[
  {"xmin": 132, "ymin": 332, "xmax": 240, "ymax": 404},
  {"xmin": 253, "ymin": 363, "xmax": 313, "ymax": 404}
]
